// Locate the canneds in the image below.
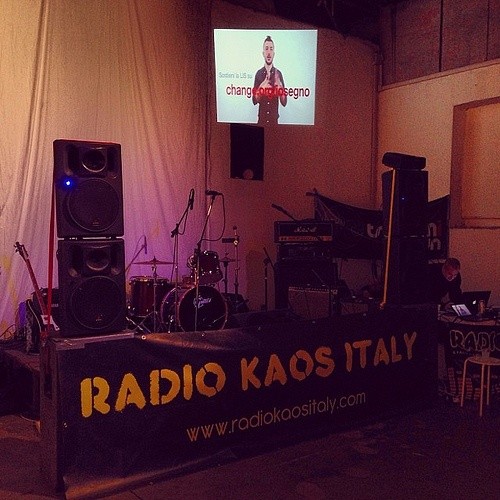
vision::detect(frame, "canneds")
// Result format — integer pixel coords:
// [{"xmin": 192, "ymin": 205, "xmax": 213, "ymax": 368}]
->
[{"xmin": 478, "ymin": 299, "xmax": 485, "ymax": 314}]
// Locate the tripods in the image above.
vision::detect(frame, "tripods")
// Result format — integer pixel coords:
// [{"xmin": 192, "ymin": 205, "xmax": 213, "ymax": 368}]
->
[{"xmin": 133, "ymin": 264, "xmax": 165, "ymax": 335}]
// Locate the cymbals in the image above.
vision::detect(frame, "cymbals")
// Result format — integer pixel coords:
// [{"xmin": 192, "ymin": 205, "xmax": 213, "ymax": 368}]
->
[
  {"xmin": 218, "ymin": 258, "xmax": 238, "ymax": 262},
  {"xmin": 132, "ymin": 260, "xmax": 176, "ymax": 264}
]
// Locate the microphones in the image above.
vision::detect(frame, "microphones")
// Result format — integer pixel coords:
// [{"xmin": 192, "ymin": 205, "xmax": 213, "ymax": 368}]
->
[
  {"xmin": 272, "ymin": 203, "xmax": 284, "ymax": 211},
  {"xmin": 233, "ymin": 225, "xmax": 237, "ymax": 241},
  {"xmin": 205, "ymin": 189, "xmax": 222, "ymax": 195},
  {"xmin": 144, "ymin": 236, "xmax": 147, "ymax": 254},
  {"xmin": 190, "ymin": 191, "xmax": 194, "ymax": 210}
]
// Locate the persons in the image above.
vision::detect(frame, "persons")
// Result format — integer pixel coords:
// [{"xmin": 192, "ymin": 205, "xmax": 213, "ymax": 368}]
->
[
  {"xmin": 428, "ymin": 258, "xmax": 463, "ymax": 310},
  {"xmin": 253, "ymin": 36, "xmax": 288, "ymax": 125}
]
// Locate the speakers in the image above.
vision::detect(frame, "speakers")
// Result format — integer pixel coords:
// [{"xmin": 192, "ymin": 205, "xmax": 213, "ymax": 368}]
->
[
  {"xmin": 382, "ymin": 166, "xmax": 449, "ymax": 306},
  {"xmin": 53, "ymin": 139, "xmax": 126, "ymax": 338},
  {"xmin": 273, "ymin": 245, "xmax": 338, "ymax": 320}
]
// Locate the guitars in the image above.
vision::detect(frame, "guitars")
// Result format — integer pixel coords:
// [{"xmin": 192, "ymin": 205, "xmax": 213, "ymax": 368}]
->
[{"xmin": 13, "ymin": 241, "xmax": 60, "ymax": 338}]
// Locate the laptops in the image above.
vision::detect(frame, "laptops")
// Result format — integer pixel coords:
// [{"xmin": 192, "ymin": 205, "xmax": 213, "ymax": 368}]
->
[
  {"xmin": 449, "ymin": 303, "xmax": 489, "ymax": 321},
  {"xmin": 461, "ymin": 290, "xmax": 492, "ymax": 315}
]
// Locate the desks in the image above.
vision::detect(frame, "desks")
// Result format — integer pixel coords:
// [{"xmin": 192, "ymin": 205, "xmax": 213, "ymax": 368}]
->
[{"xmin": 437, "ymin": 314, "xmax": 500, "ymax": 404}]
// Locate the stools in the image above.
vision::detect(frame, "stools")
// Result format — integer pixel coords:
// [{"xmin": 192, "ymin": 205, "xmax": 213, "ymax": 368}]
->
[{"xmin": 460, "ymin": 355, "xmax": 500, "ymax": 416}]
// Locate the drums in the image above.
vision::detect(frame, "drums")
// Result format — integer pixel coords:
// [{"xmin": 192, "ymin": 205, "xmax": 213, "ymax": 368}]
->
[
  {"xmin": 130, "ymin": 276, "xmax": 168, "ymax": 322},
  {"xmin": 160, "ymin": 284, "xmax": 227, "ymax": 333},
  {"xmin": 188, "ymin": 251, "xmax": 223, "ymax": 283}
]
